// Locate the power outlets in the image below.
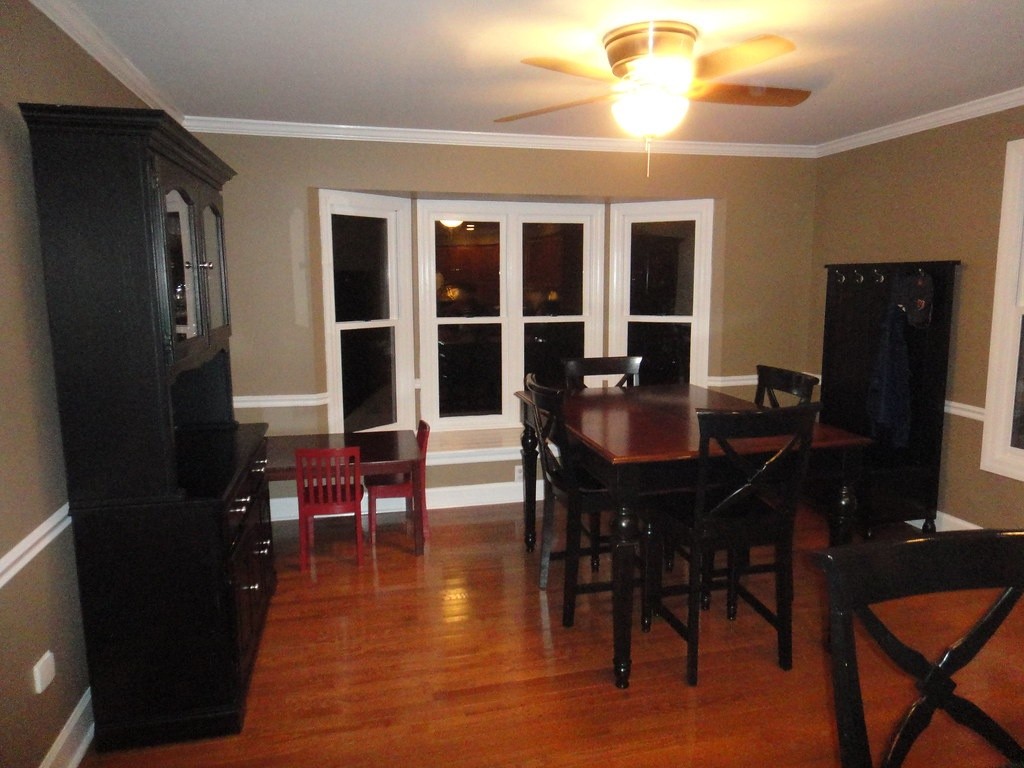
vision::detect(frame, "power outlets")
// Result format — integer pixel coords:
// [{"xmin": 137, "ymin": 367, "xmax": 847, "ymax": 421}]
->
[{"xmin": 34, "ymin": 650, "xmax": 56, "ymax": 695}]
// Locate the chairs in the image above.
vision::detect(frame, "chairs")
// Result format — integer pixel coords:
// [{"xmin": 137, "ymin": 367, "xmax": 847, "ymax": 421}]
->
[
  {"xmin": 641, "ymin": 401, "xmax": 826, "ymax": 687},
  {"xmin": 524, "ymin": 373, "xmax": 664, "ymax": 628},
  {"xmin": 560, "ymin": 354, "xmax": 644, "ymax": 571},
  {"xmin": 664, "ymin": 364, "xmax": 821, "ymax": 610},
  {"xmin": 364, "ymin": 419, "xmax": 430, "ymax": 544},
  {"xmin": 295, "ymin": 447, "xmax": 366, "ymax": 573},
  {"xmin": 806, "ymin": 529, "xmax": 1024, "ymax": 768}
]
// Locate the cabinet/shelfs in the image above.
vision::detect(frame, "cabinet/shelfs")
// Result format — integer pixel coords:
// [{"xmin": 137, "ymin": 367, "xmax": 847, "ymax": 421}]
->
[{"xmin": 18, "ymin": 100, "xmax": 279, "ymax": 756}]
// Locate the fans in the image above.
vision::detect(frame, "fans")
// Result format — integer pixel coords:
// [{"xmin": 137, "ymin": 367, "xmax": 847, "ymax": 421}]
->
[{"xmin": 494, "ymin": 20, "xmax": 813, "ymax": 123}]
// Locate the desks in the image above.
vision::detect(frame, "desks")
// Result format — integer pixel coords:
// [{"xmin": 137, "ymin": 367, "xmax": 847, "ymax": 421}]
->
[
  {"xmin": 265, "ymin": 430, "xmax": 424, "ymax": 556},
  {"xmin": 516, "ymin": 382, "xmax": 874, "ymax": 689}
]
